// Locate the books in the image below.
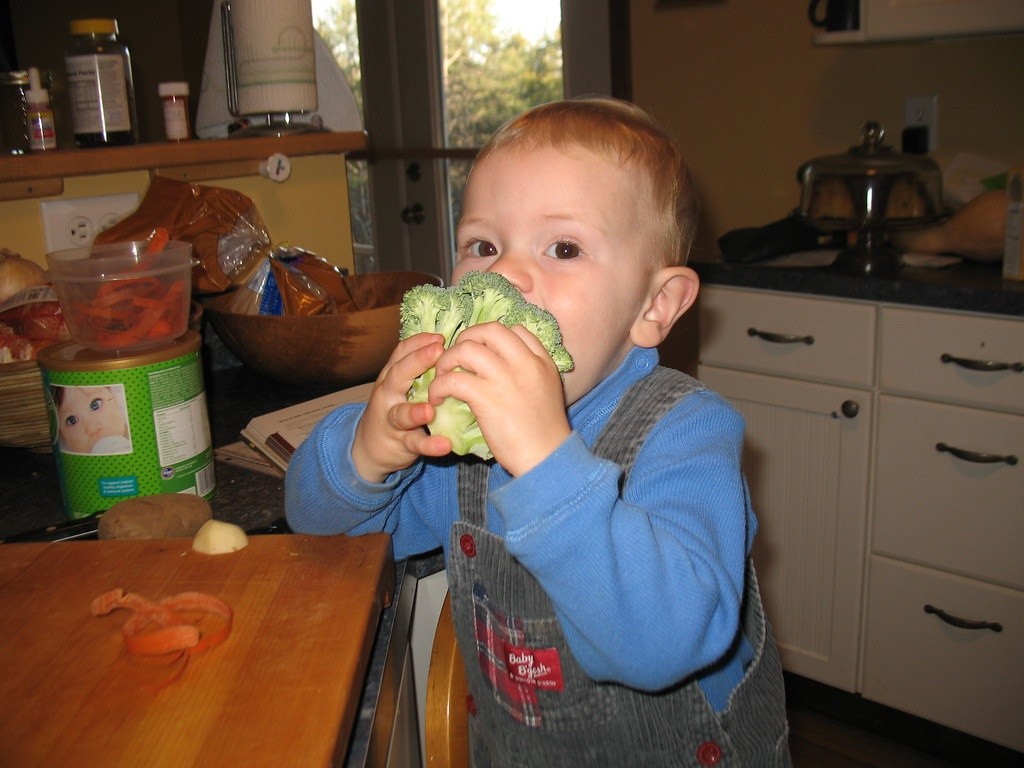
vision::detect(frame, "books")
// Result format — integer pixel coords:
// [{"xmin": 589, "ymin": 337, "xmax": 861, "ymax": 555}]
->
[{"xmin": 240, "ymin": 381, "xmax": 378, "ymax": 472}]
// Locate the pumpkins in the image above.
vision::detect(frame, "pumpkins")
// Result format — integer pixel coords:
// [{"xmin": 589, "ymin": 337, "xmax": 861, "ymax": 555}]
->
[{"xmin": 892, "ymin": 182, "xmax": 1008, "ymax": 259}]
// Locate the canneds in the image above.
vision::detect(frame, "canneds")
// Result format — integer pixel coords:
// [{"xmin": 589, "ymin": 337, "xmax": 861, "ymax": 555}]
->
[
  {"xmin": 36, "ymin": 332, "xmax": 219, "ymax": 520},
  {"xmin": 0, "ymin": 69, "xmax": 54, "ymax": 153}
]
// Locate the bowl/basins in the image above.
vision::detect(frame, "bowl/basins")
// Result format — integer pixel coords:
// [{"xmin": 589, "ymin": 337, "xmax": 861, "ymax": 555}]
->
[
  {"xmin": 46, "ymin": 240, "xmax": 198, "ymax": 351},
  {"xmin": 203, "ymin": 270, "xmax": 443, "ymax": 379}
]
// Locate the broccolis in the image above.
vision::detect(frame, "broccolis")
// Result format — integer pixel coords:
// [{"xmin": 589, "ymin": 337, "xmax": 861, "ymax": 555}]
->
[{"xmin": 392, "ymin": 272, "xmax": 572, "ymax": 454}]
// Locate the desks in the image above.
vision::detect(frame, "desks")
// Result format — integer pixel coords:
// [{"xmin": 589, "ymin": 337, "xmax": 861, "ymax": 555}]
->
[{"xmin": 0, "ymin": 533, "xmax": 398, "ymax": 768}]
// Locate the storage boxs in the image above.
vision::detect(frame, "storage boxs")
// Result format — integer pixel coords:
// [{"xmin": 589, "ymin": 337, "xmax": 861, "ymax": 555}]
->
[{"xmin": 1001, "ymin": 168, "xmax": 1024, "ymax": 281}]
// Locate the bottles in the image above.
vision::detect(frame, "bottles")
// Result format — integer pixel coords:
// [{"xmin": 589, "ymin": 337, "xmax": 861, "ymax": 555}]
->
[
  {"xmin": 24, "ymin": 66, "xmax": 56, "ymax": 151},
  {"xmin": 68, "ymin": 19, "xmax": 138, "ymax": 145},
  {"xmin": 159, "ymin": 82, "xmax": 190, "ymax": 142}
]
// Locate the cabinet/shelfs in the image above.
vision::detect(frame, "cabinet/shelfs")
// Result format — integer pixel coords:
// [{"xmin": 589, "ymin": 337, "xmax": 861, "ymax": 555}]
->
[
  {"xmin": 692, "ymin": 250, "xmax": 1024, "ymax": 768},
  {"xmin": 0, "ymin": 131, "xmax": 370, "ymax": 276}
]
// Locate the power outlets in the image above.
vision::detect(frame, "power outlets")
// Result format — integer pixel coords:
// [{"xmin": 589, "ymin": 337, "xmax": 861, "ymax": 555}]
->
[
  {"xmin": 906, "ymin": 93, "xmax": 941, "ymax": 152},
  {"xmin": 45, "ymin": 191, "xmax": 142, "ymax": 262}
]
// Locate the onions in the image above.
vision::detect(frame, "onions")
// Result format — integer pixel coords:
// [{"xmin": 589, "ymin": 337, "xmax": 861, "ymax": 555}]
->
[{"xmin": 0, "ymin": 253, "xmax": 45, "ymax": 304}]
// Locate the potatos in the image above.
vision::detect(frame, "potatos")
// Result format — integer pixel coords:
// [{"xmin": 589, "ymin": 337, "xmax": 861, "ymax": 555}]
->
[{"xmin": 97, "ymin": 493, "xmax": 212, "ymax": 540}]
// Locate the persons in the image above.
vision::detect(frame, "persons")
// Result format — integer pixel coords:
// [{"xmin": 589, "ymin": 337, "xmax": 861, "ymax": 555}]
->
[
  {"xmin": 52, "ymin": 386, "xmax": 129, "ymax": 454},
  {"xmin": 285, "ymin": 99, "xmax": 790, "ymax": 768}
]
